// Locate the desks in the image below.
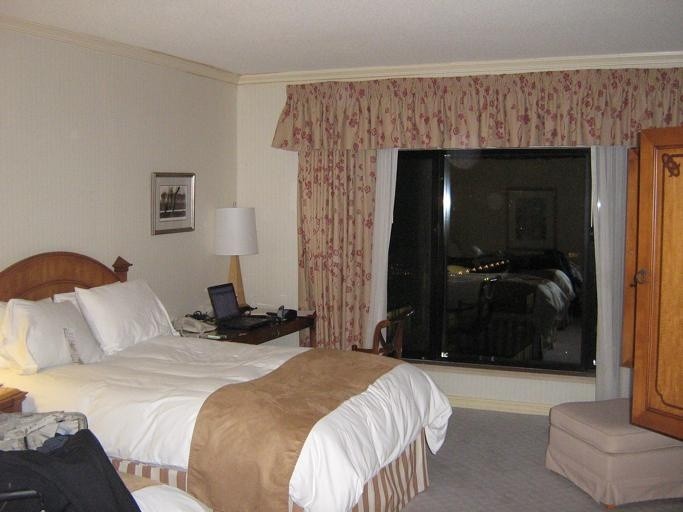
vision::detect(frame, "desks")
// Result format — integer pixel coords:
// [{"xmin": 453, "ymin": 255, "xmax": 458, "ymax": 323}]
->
[{"xmin": 207, "ymin": 309, "xmax": 316, "ymax": 356}]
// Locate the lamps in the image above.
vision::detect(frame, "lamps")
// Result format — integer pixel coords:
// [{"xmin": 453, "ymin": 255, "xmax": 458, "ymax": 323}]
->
[{"xmin": 213, "ymin": 208, "xmax": 258, "ymax": 308}]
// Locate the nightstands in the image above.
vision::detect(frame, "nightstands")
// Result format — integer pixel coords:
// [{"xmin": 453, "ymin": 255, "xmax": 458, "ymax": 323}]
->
[{"xmin": 0, "ymin": 386, "xmax": 28, "ymax": 415}]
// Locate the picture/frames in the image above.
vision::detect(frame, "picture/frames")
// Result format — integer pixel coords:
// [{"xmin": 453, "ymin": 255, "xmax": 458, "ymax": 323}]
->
[
  {"xmin": 150, "ymin": 173, "xmax": 196, "ymax": 235},
  {"xmin": 506, "ymin": 188, "xmax": 556, "ymax": 251}
]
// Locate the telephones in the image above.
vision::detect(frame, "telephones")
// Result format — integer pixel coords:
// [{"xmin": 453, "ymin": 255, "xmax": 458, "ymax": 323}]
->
[{"xmin": 277, "ymin": 305, "xmax": 297, "ymax": 321}]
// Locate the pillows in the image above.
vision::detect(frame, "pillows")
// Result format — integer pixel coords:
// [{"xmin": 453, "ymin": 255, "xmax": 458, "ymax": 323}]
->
[
  {"xmin": 0, "ymin": 298, "xmax": 52, "ymax": 357},
  {"xmin": 74, "ymin": 278, "xmax": 181, "ymax": 355},
  {"xmin": 12, "ymin": 300, "xmax": 105, "ymax": 372},
  {"xmin": 53, "ymin": 292, "xmax": 79, "ymax": 307}
]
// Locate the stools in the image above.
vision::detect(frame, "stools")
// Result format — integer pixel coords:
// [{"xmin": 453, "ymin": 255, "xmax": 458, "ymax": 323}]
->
[{"xmin": 545, "ymin": 399, "xmax": 683, "ymax": 508}]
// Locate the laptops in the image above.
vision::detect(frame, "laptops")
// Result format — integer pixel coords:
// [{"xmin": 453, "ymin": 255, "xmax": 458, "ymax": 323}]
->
[{"xmin": 207, "ymin": 282, "xmax": 268, "ymax": 331}]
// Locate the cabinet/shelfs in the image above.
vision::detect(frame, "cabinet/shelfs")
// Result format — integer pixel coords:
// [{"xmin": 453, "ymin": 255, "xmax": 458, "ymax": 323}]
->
[{"xmin": 622, "ymin": 128, "xmax": 683, "ymax": 441}]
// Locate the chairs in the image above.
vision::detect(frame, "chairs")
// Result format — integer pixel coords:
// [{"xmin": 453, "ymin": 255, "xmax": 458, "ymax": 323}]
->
[{"xmin": 352, "ymin": 308, "xmax": 416, "ymax": 359}]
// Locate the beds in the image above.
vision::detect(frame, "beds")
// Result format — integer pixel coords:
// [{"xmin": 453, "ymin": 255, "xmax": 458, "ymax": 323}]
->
[
  {"xmin": 525, "ymin": 269, "xmax": 576, "ymax": 302},
  {"xmin": 442, "ymin": 273, "xmax": 569, "ymax": 360},
  {"xmin": 0, "ymin": 252, "xmax": 452, "ymax": 512},
  {"xmin": 117, "ymin": 471, "xmax": 214, "ymax": 512}
]
